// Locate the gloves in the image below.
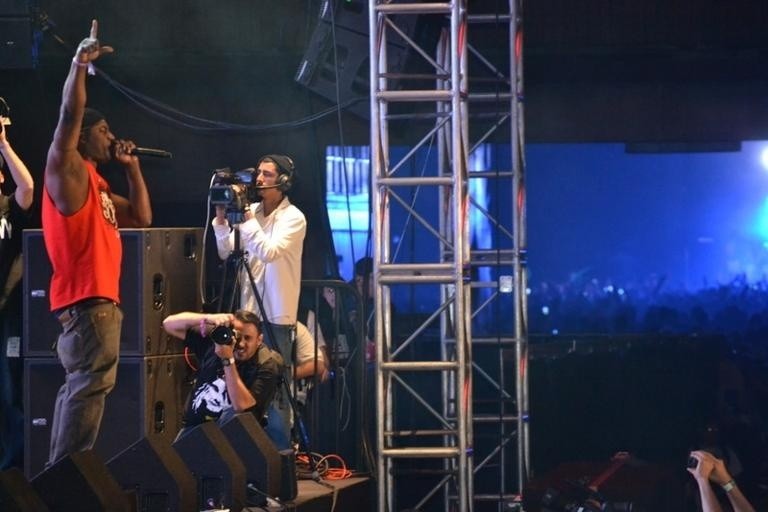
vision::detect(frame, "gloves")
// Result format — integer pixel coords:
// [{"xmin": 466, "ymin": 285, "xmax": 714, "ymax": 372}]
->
[{"xmin": 230, "ymin": 183, "xmax": 251, "ymax": 215}]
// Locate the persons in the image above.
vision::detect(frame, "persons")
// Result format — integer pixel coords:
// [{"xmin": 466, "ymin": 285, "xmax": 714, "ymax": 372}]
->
[
  {"xmin": 211, "ymin": 154, "xmax": 307, "ymax": 450},
  {"xmin": 162, "ymin": 307, "xmax": 280, "ymax": 441},
  {"xmin": 294, "ymin": 255, "xmax": 767, "ymax": 431},
  {"xmin": 0, "ymin": 96, "xmax": 34, "ymax": 418},
  {"xmin": 40, "ymin": 18, "xmax": 154, "ymax": 472},
  {"xmin": 686, "ymin": 449, "xmax": 755, "ymax": 512}
]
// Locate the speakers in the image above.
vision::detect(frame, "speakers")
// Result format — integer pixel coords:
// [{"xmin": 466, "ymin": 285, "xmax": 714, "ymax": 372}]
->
[
  {"xmin": 22, "ymin": 354, "xmax": 200, "ymax": 482},
  {"xmin": 21, "ymin": 227, "xmax": 221, "ymax": 358}
]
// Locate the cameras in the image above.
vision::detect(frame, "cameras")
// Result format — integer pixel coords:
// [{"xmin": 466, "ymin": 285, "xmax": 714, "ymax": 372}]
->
[{"xmin": 210, "ymin": 321, "xmax": 237, "ymax": 347}]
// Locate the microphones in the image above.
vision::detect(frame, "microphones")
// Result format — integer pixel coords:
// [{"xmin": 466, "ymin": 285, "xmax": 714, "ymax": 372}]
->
[
  {"xmin": 253, "ymin": 182, "xmax": 282, "ymax": 190},
  {"xmin": 111, "ymin": 146, "xmax": 174, "ymax": 160}
]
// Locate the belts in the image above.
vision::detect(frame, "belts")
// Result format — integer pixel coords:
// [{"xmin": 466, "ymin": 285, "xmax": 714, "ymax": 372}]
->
[{"xmin": 69, "ymin": 298, "xmax": 112, "ymax": 315}]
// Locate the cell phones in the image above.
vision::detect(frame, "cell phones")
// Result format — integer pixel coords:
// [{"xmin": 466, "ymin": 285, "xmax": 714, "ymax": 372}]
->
[{"xmin": 689, "ymin": 456, "xmax": 698, "ymax": 469}]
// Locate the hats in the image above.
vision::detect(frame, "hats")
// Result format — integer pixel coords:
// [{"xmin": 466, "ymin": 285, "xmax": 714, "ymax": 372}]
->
[{"xmin": 255, "ymin": 154, "xmax": 295, "ymax": 177}]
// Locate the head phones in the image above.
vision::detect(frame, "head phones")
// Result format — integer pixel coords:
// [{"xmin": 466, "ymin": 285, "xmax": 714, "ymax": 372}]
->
[{"xmin": 274, "ymin": 155, "xmax": 295, "ymax": 192}]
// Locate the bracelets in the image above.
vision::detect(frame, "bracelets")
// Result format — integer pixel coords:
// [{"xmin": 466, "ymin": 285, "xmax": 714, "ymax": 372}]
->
[
  {"xmin": 72, "ymin": 60, "xmax": 89, "ymax": 67},
  {"xmin": 722, "ymin": 478, "xmax": 736, "ymax": 492}
]
